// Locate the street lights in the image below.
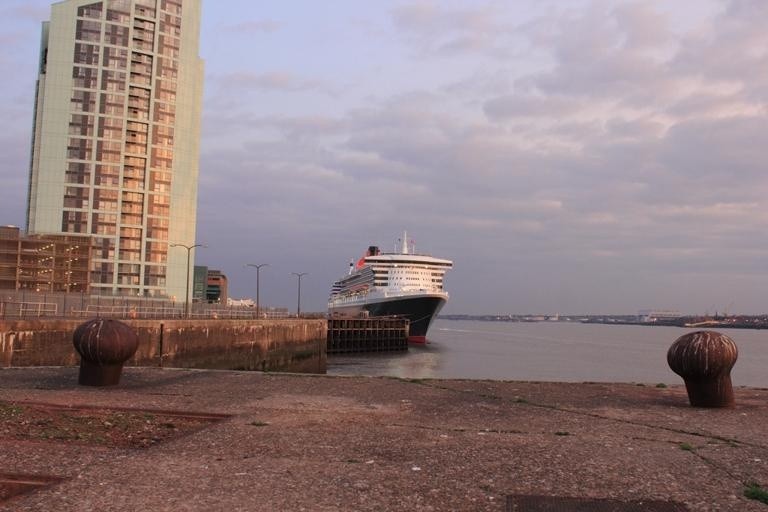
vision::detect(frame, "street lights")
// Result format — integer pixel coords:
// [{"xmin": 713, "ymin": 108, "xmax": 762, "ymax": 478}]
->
[
  {"xmin": 170, "ymin": 243, "xmax": 209, "ymax": 319},
  {"xmin": 243, "ymin": 262, "xmax": 271, "ymax": 320},
  {"xmin": 287, "ymin": 272, "xmax": 309, "ymax": 318}
]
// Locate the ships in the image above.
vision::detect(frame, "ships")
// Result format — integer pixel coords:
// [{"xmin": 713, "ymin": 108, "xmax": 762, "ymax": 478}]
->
[{"xmin": 328, "ymin": 229, "xmax": 455, "ymax": 346}]
